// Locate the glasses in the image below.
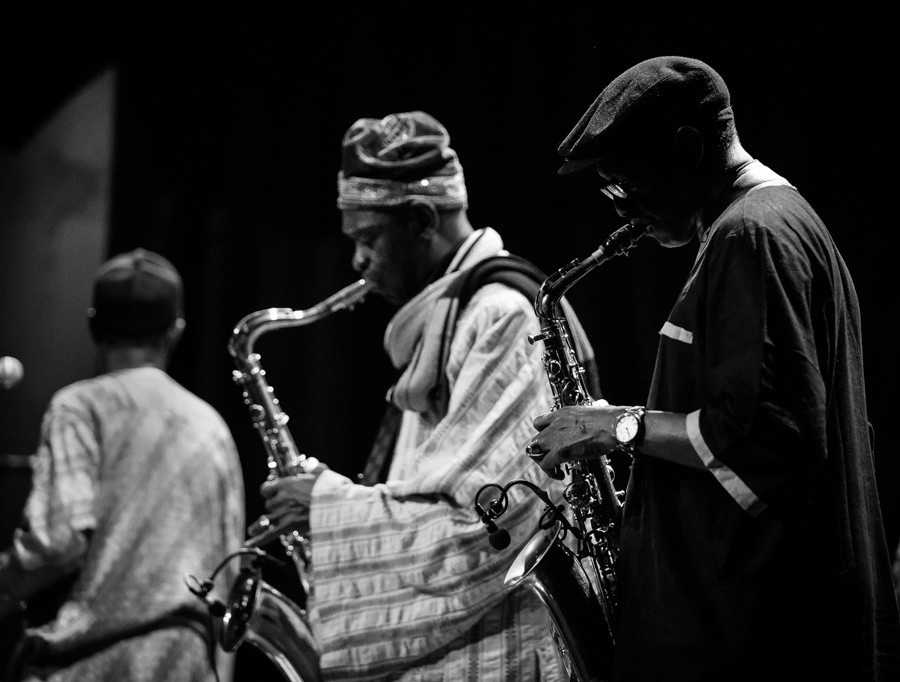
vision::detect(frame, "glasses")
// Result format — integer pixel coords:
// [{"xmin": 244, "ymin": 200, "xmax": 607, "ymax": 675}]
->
[{"xmin": 600, "ymin": 150, "xmax": 686, "ymax": 202}]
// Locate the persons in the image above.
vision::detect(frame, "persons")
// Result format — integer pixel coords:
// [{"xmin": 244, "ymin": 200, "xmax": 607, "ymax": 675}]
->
[
  {"xmin": 1, "ymin": 248, "xmax": 247, "ymax": 682},
  {"xmin": 239, "ymin": 113, "xmax": 598, "ymax": 680},
  {"xmin": 528, "ymin": 54, "xmax": 896, "ymax": 682}
]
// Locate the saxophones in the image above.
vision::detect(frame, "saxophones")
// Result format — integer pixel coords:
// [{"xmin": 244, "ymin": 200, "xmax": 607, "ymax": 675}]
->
[
  {"xmin": 502, "ymin": 216, "xmax": 653, "ymax": 681},
  {"xmin": 216, "ymin": 271, "xmax": 380, "ymax": 681}
]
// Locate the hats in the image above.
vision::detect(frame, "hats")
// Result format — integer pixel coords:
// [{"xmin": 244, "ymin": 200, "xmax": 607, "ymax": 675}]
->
[
  {"xmin": 91, "ymin": 248, "xmax": 183, "ymax": 334},
  {"xmin": 336, "ymin": 111, "xmax": 468, "ymax": 214},
  {"xmin": 556, "ymin": 59, "xmax": 731, "ymax": 177}
]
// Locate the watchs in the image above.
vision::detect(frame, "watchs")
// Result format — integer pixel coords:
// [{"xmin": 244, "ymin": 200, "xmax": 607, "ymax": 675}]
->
[{"xmin": 612, "ymin": 405, "xmax": 646, "ymax": 458}]
